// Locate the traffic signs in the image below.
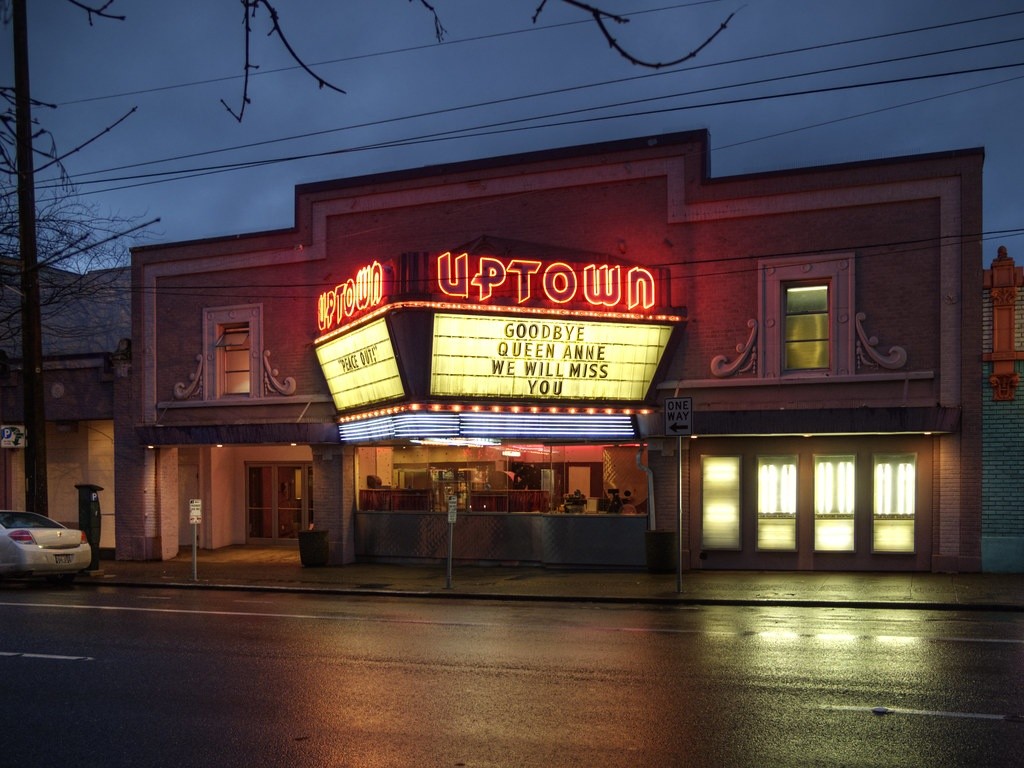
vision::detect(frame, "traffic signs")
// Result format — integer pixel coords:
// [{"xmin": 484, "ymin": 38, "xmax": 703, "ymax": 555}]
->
[{"xmin": 663, "ymin": 396, "xmax": 693, "ymax": 438}]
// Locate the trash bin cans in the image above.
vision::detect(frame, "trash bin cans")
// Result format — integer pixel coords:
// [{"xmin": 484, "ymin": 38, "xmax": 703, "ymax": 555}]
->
[
  {"xmin": 645, "ymin": 530, "xmax": 679, "ymax": 576},
  {"xmin": 298, "ymin": 529, "xmax": 329, "ymax": 567}
]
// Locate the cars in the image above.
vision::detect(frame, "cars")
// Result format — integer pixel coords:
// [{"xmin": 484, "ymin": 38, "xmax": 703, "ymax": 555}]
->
[{"xmin": 0, "ymin": 510, "xmax": 93, "ymax": 576}]
